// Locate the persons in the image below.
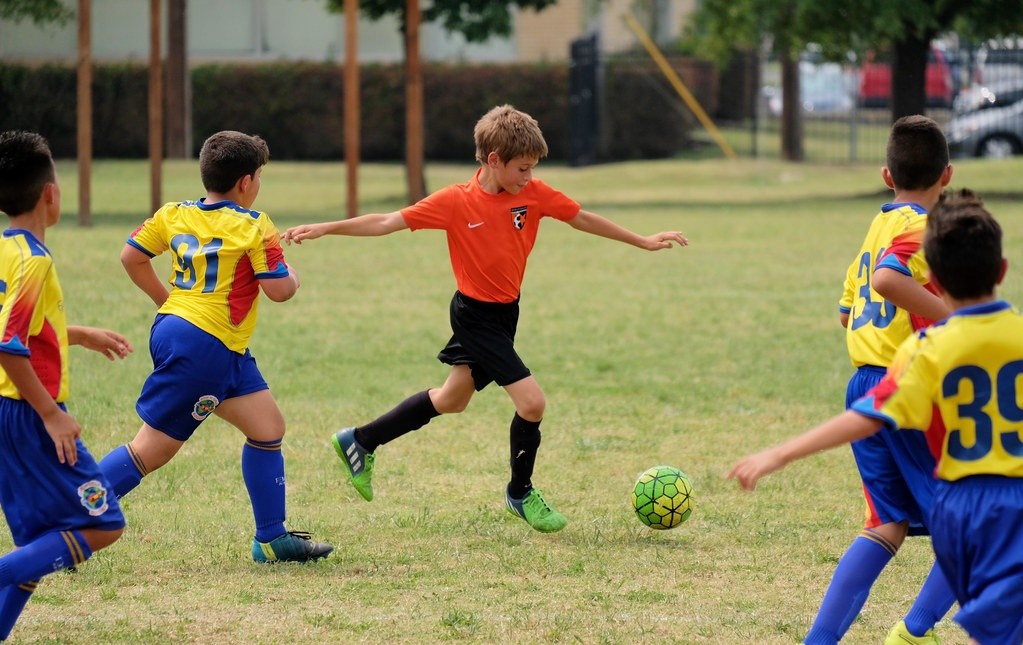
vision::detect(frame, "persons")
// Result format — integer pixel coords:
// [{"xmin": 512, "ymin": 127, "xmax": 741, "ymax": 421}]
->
[
  {"xmin": 803, "ymin": 116, "xmax": 957, "ymax": 645},
  {"xmin": 61, "ymin": 131, "xmax": 333, "ymax": 573},
  {"xmin": 725, "ymin": 188, "xmax": 1023, "ymax": 645},
  {"xmin": 279, "ymin": 105, "xmax": 688, "ymax": 533},
  {"xmin": 0, "ymin": 131, "xmax": 133, "ymax": 642}
]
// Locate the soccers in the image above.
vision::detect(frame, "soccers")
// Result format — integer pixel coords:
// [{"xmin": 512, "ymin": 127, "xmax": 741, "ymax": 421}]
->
[{"xmin": 631, "ymin": 466, "xmax": 696, "ymax": 531}]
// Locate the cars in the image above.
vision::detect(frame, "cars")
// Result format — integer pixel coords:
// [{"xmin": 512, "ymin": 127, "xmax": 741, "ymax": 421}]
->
[
  {"xmin": 860, "ymin": 46, "xmax": 954, "ymax": 108},
  {"xmin": 971, "ymin": 39, "xmax": 1023, "ymax": 84},
  {"xmin": 946, "ymin": 99, "xmax": 1023, "ymax": 160},
  {"xmin": 953, "ymin": 78, "xmax": 1023, "ymax": 116}
]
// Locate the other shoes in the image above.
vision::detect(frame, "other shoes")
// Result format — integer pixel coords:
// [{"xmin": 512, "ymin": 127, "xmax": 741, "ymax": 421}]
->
[{"xmin": 885, "ymin": 621, "xmax": 939, "ymax": 645}]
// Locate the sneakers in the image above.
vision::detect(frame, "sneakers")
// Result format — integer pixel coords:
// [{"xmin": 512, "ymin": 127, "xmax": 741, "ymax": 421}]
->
[
  {"xmin": 506, "ymin": 481, "xmax": 568, "ymax": 534},
  {"xmin": 332, "ymin": 427, "xmax": 374, "ymax": 502},
  {"xmin": 251, "ymin": 531, "xmax": 335, "ymax": 563}
]
